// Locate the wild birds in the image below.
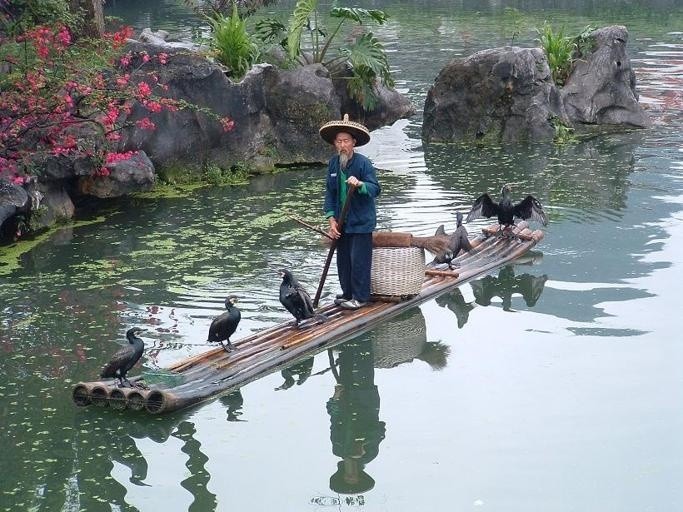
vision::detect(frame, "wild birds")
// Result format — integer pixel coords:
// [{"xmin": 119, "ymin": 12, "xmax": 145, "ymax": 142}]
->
[
  {"xmin": 469, "ymin": 265, "xmax": 548, "ymax": 313},
  {"xmin": 110, "ymin": 435, "xmax": 152, "ymax": 487},
  {"xmin": 435, "ymin": 288, "xmax": 478, "ymax": 329},
  {"xmin": 431, "ymin": 212, "xmax": 482, "ymax": 270},
  {"xmin": 219, "ymin": 388, "xmax": 247, "ymax": 422},
  {"xmin": 274, "ymin": 356, "xmax": 314, "ymax": 392},
  {"xmin": 464, "ymin": 184, "xmax": 549, "ymax": 236},
  {"xmin": 276, "ymin": 269, "xmax": 317, "ymax": 326},
  {"xmin": 99, "ymin": 327, "xmax": 148, "ymax": 390},
  {"xmin": 207, "ymin": 295, "xmax": 241, "ymax": 353}
]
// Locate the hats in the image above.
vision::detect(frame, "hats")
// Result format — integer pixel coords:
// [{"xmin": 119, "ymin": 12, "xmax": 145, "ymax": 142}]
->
[{"xmin": 319, "ymin": 114, "xmax": 370, "ymax": 147}]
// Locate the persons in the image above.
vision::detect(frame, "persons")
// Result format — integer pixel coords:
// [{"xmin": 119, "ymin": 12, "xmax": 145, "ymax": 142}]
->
[
  {"xmin": 322, "ymin": 338, "xmax": 387, "ymax": 497},
  {"xmin": 315, "ymin": 111, "xmax": 380, "ymax": 312}
]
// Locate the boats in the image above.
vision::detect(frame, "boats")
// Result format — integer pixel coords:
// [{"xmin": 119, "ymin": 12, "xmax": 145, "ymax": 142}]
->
[{"xmin": 70, "ymin": 220, "xmax": 548, "ymax": 413}]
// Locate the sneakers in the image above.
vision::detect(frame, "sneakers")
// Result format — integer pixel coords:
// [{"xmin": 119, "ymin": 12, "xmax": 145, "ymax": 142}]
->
[{"xmin": 334, "ymin": 298, "xmax": 370, "ymax": 310}]
